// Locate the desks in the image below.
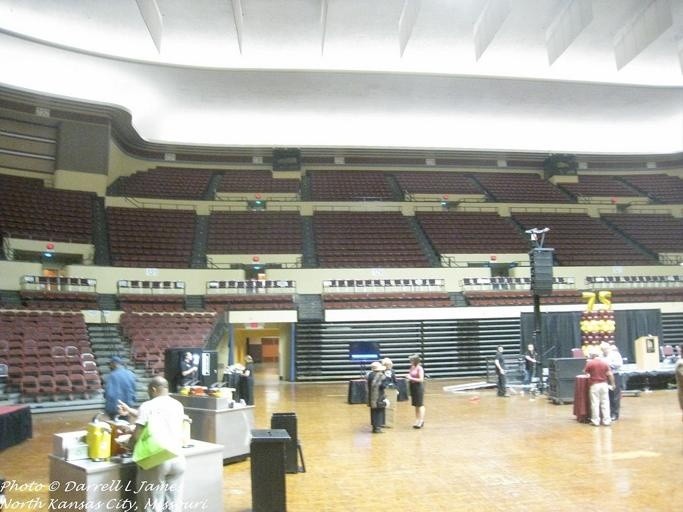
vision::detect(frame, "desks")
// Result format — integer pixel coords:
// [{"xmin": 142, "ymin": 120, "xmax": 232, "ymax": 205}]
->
[
  {"xmin": 0, "ymin": 405, "xmax": 32, "ymax": 452},
  {"xmin": 46, "ymin": 437, "xmax": 226, "ymax": 512},
  {"xmin": 166, "ymin": 387, "xmax": 255, "ymax": 467},
  {"xmin": 573, "ymin": 373, "xmax": 590, "ymax": 421}
]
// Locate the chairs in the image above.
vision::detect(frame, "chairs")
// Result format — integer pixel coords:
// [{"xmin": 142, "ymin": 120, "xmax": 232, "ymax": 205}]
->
[{"xmin": 2, "ymin": 165, "xmax": 683, "ymax": 404}]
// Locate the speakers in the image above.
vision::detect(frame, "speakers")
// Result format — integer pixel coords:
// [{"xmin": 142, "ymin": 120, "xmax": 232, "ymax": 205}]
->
[
  {"xmin": 165, "ymin": 347, "xmax": 202, "ymax": 393},
  {"xmin": 271, "ymin": 413, "xmax": 298, "ymax": 474},
  {"xmin": 548, "ymin": 358, "xmax": 587, "ymax": 405},
  {"xmin": 528, "ymin": 247, "xmax": 554, "ymax": 295},
  {"xmin": 349, "ymin": 380, "xmax": 369, "ymax": 404},
  {"xmin": 201, "ymin": 351, "xmax": 218, "ymax": 387},
  {"xmin": 395, "ymin": 378, "xmax": 408, "ymax": 401},
  {"xmin": 249, "ymin": 429, "xmax": 291, "ymax": 512}
]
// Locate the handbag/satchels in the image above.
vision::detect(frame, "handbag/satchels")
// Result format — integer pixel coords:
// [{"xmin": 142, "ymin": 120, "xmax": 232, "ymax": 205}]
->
[{"xmin": 132, "ymin": 422, "xmax": 178, "ymax": 470}]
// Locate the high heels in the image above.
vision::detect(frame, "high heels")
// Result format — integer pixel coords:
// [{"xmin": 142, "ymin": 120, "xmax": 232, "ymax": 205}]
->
[{"xmin": 413, "ymin": 420, "xmax": 425, "ymax": 429}]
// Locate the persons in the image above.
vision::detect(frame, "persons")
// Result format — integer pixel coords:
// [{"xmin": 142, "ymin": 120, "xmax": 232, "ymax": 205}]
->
[
  {"xmin": 117, "ymin": 376, "xmax": 185, "ymax": 512},
  {"xmin": 240, "ymin": 355, "xmax": 254, "ymax": 405},
  {"xmin": 406, "ymin": 355, "xmax": 425, "ymax": 429},
  {"xmin": 583, "ymin": 342, "xmax": 623, "ymax": 426},
  {"xmin": 523, "ymin": 343, "xmax": 537, "ymax": 391},
  {"xmin": 103, "ymin": 356, "xmax": 138, "ymax": 420},
  {"xmin": 367, "ymin": 358, "xmax": 400, "ymax": 433},
  {"xmin": 675, "ymin": 345, "xmax": 683, "ymax": 410},
  {"xmin": 179, "ymin": 351, "xmax": 198, "ymax": 386},
  {"xmin": 494, "ymin": 346, "xmax": 508, "ymax": 397}
]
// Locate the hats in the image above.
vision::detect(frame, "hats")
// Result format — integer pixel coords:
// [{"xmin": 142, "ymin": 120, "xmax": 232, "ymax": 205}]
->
[
  {"xmin": 106, "ymin": 356, "xmax": 126, "ymax": 365},
  {"xmin": 244, "ymin": 355, "xmax": 253, "ymax": 363},
  {"xmin": 370, "ymin": 361, "xmax": 386, "ymax": 372}
]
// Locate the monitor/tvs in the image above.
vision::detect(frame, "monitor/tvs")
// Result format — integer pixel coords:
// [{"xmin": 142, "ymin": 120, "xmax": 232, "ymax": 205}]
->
[{"xmin": 350, "ymin": 342, "xmax": 380, "ymax": 360}]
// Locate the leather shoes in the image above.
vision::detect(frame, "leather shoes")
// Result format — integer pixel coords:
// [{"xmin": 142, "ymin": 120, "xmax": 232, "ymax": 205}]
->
[{"xmin": 372, "ymin": 428, "xmax": 382, "ymax": 433}]
